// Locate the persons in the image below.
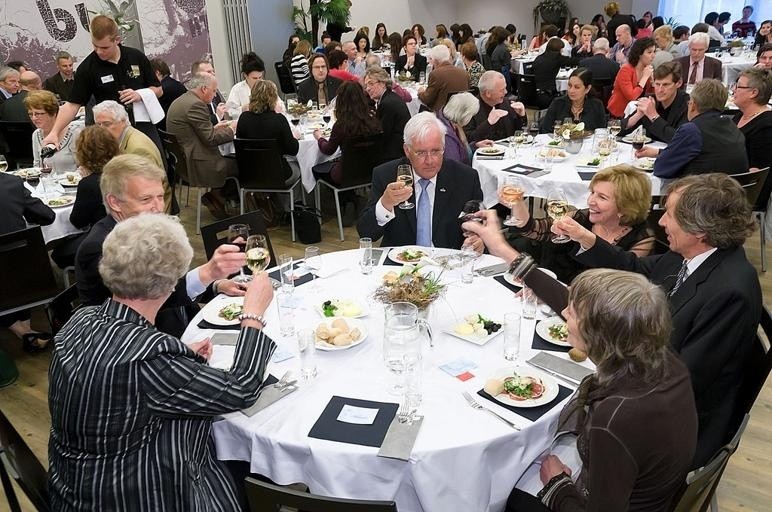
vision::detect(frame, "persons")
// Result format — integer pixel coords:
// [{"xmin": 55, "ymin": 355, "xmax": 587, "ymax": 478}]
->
[
  {"xmin": 2, "ymin": 4, "xmax": 771, "ymax": 350},
  {"xmin": 47, "ymin": 211, "xmax": 274, "ymax": 511},
  {"xmin": 549, "ymin": 173, "xmax": 763, "ymax": 470},
  {"xmin": 461, "ymin": 208, "xmax": 701, "ymax": 511}
]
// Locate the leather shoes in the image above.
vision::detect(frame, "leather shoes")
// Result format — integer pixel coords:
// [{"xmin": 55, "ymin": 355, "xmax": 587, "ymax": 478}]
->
[
  {"xmin": 280, "ymin": 211, "xmax": 297, "ymax": 227},
  {"xmin": 201, "ymin": 192, "xmax": 231, "ymax": 220},
  {"xmin": 342, "ymin": 200, "xmax": 355, "ymax": 227}
]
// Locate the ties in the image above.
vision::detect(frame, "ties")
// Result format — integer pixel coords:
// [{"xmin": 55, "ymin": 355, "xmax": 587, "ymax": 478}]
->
[
  {"xmin": 669, "ymin": 260, "xmax": 688, "ymax": 297},
  {"xmin": 318, "ymin": 82, "xmax": 326, "ymax": 106},
  {"xmin": 689, "ymin": 61, "xmax": 699, "ymax": 84},
  {"xmin": 416, "ymin": 179, "xmax": 431, "ymax": 248}
]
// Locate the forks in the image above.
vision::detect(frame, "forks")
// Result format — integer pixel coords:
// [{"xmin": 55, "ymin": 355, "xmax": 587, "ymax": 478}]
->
[
  {"xmin": 260, "ymin": 370, "xmax": 293, "ymax": 394},
  {"xmin": 461, "ymin": 390, "xmax": 522, "ymax": 432}
]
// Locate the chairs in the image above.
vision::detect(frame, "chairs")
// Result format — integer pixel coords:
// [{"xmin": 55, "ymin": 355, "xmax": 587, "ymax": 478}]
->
[
  {"xmin": 659, "ymin": 413, "xmax": 751, "ymax": 512},
  {"xmin": 245, "ymin": 476, "xmax": 398, "ymax": 511},
  {"xmin": 0, "ymin": 415, "xmax": 47, "ymax": 512}
]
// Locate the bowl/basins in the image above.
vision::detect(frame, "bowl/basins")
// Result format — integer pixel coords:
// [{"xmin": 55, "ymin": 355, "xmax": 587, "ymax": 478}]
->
[{"xmin": 565, "ymin": 139, "xmax": 583, "ymax": 154}]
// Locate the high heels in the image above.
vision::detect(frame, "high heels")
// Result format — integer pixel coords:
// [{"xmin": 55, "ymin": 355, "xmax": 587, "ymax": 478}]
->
[{"xmin": 21, "ymin": 332, "xmax": 51, "ymax": 351}]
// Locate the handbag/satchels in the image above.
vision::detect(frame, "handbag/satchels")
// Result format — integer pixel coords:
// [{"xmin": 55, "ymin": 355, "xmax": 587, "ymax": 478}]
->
[
  {"xmin": 245, "ymin": 191, "xmax": 283, "ymax": 228},
  {"xmin": 296, "ymin": 208, "xmax": 323, "ymax": 244}
]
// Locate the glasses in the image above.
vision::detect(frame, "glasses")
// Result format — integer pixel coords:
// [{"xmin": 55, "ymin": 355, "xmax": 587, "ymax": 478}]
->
[
  {"xmin": 643, "ymin": 15, "xmax": 650, "ymax": 18},
  {"xmin": 651, "ymin": 82, "xmax": 677, "ymax": 88},
  {"xmin": 312, "ymin": 65, "xmax": 327, "ymax": 69},
  {"xmin": 407, "ymin": 144, "xmax": 445, "ymax": 159},
  {"xmin": 364, "ymin": 81, "xmax": 382, "ymax": 88},
  {"xmin": 4, "ymin": 79, "xmax": 21, "ymax": 84},
  {"xmin": 732, "ymin": 81, "xmax": 756, "ymax": 90},
  {"xmin": 685, "ymin": 33, "xmax": 691, "ymax": 36},
  {"xmin": 406, "ymin": 42, "xmax": 416, "ymax": 45},
  {"xmin": 27, "ymin": 111, "xmax": 49, "ymax": 118}
]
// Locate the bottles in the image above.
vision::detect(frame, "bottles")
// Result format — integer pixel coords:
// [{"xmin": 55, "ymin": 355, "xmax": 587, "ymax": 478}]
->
[
  {"xmin": 419, "ymin": 71, "xmax": 424, "ymax": 85},
  {"xmin": 746, "ymin": 30, "xmax": 752, "ymax": 39},
  {"xmin": 395, "ymin": 71, "xmax": 400, "ymax": 85},
  {"xmin": 426, "ymin": 39, "xmax": 430, "ymax": 51},
  {"xmin": 40, "ymin": 141, "xmax": 62, "ymax": 159},
  {"xmin": 284, "ymin": 93, "xmax": 298, "ymax": 115},
  {"xmin": 554, "ymin": 120, "xmax": 561, "ymax": 140}
]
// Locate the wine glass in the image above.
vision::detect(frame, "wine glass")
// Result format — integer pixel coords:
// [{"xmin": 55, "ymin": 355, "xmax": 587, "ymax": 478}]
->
[
  {"xmin": 564, "ymin": 117, "xmax": 572, "ymax": 125},
  {"xmin": 245, "ymin": 235, "xmax": 270, "ymax": 279},
  {"xmin": 599, "ymin": 142, "xmax": 610, "ymax": 169},
  {"xmin": 323, "ymin": 112, "xmax": 332, "ymax": 128},
  {"xmin": 501, "ymin": 176, "xmax": 524, "ymax": 227},
  {"xmin": 0, "ymin": 155, "xmax": 8, "ymax": 173},
  {"xmin": 39, "ymin": 160, "xmax": 53, "ymax": 177},
  {"xmin": 396, "ymin": 165, "xmax": 415, "ymax": 209},
  {"xmin": 290, "ymin": 113, "xmax": 300, "ymax": 130},
  {"xmin": 530, "ymin": 122, "xmax": 539, "ymax": 146},
  {"xmin": 609, "ymin": 119, "xmax": 621, "ymax": 140},
  {"xmin": 331, "ymin": 107, "xmax": 337, "ymax": 123},
  {"xmin": 304, "ymin": 246, "xmax": 324, "ymax": 294},
  {"xmin": 610, "ymin": 142, "xmax": 620, "ymax": 165},
  {"xmin": 299, "ymin": 115, "xmax": 307, "ymax": 131},
  {"xmin": 631, "ymin": 129, "xmax": 645, "ymax": 163},
  {"xmin": 513, "ymin": 131, "xmax": 523, "ymax": 157},
  {"xmin": 533, "ymin": 137, "xmax": 542, "ymax": 163},
  {"xmin": 227, "ymin": 225, "xmax": 254, "ymax": 285},
  {"xmin": 26, "ymin": 170, "xmax": 41, "ymax": 198}
]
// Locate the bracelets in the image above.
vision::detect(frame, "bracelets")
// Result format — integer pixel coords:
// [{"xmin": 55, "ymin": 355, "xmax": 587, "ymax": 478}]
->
[
  {"xmin": 508, "ymin": 252, "xmax": 538, "ymax": 280},
  {"xmin": 234, "ymin": 312, "xmax": 270, "ymax": 327}
]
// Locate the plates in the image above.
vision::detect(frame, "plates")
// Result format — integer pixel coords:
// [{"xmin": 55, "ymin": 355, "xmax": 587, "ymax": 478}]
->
[
  {"xmin": 387, "ymin": 245, "xmax": 434, "ymax": 265},
  {"xmin": 314, "ymin": 297, "xmax": 371, "ymax": 319},
  {"xmin": 59, "ymin": 180, "xmax": 80, "ymax": 186},
  {"xmin": 209, "ymin": 346, "xmax": 235, "ymax": 371},
  {"xmin": 542, "ymin": 156, "xmax": 568, "ymax": 163},
  {"xmin": 622, "ymin": 137, "xmax": 652, "ymax": 144},
  {"xmin": 545, "ymin": 139, "xmax": 566, "ymax": 149},
  {"xmin": 17, "ymin": 168, "xmax": 42, "ymax": 178},
  {"xmin": 536, "ymin": 315, "xmax": 572, "ymax": 347},
  {"xmin": 314, "ymin": 317, "xmax": 369, "ymax": 350},
  {"xmin": 505, "ymin": 268, "xmax": 557, "ymax": 288},
  {"xmin": 507, "ymin": 135, "xmax": 533, "ymax": 144},
  {"xmin": 633, "ymin": 159, "xmax": 657, "ymax": 170},
  {"xmin": 494, "ymin": 374, "xmax": 559, "ymax": 407},
  {"xmin": 59, "ymin": 173, "xmax": 60, "ymax": 174},
  {"xmin": 441, "ymin": 320, "xmax": 504, "ymax": 345},
  {"xmin": 476, "ymin": 147, "xmax": 505, "ymax": 155},
  {"xmin": 203, "ymin": 299, "xmax": 241, "ymax": 326},
  {"xmin": 44, "ymin": 196, "xmax": 76, "ymax": 208}
]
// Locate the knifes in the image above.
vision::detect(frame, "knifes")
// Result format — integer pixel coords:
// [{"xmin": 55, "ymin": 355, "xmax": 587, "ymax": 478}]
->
[
  {"xmin": 525, "ymin": 360, "xmax": 580, "ymax": 389},
  {"xmin": 279, "ymin": 380, "xmax": 298, "ymax": 393}
]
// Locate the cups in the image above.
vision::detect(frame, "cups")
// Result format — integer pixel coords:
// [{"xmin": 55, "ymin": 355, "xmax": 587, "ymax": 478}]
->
[
  {"xmin": 715, "ymin": 49, "xmax": 719, "ymax": 57},
  {"xmin": 312, "ymin": 102, "xmax": 317, "ymax": 112},
  {"xmin": 397, "ymin": 350, "xmax": 421, "ymax": 426},
  {"xmin": 278, "ymin": 254, "xmax": 295, "ymax": 294},
  {"xmin": 276, "ymin": 292, "xmax": 296, "ymax": 337},
  {"xmin": 296, "ymin": 329, "xmax": 317, "ymax": 380},
  {"xmin": 522, "ymin": 282, "xmax": 537, "ymax": 320},
  {"xmin": 504, "ymin": 313, "xmax": 521, "ymax": 361},
  {"xmin": 40, "ymin": 173, "xmax": 57, "ymax": 193},
  {"xmin": 462, "ymin": 258, "xmax": 474, "ymax": 283},
  {"xmin": 359, "ymin": 237, "xmax": 373, "ymax": 274},
  {"xmin": 319, "ymin": 98, "xmax": 326, "ymax": 111},
  {"xmin": 384, "ymin": 302, "xmax": 431, "ymax": 375},
  {"xmin": 544, "ymin": 147, "xmax": 553, "ymax": 173},
  {"xmin": 509, "ymin": 136, "xmax": 519, "ymax": 159}
]
